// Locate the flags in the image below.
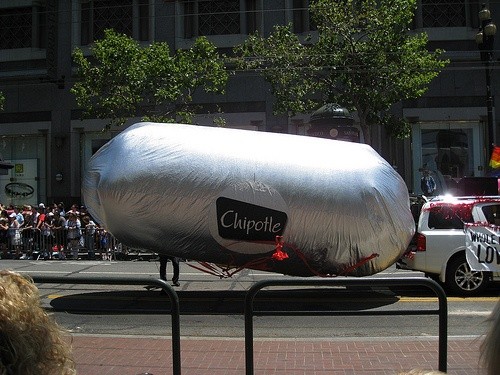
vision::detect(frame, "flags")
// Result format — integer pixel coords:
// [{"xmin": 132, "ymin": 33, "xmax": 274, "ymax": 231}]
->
[
  {"xmin": 463, "ymin": 223, "xmax": 500, "ymax": 272},
  {"xmin": 486, "ymin": 147, "xmax": 500, "ymax": 173}
]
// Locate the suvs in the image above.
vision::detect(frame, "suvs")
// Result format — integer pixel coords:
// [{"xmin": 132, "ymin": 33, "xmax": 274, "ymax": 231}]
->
[{"xmin": 396, "ymin": 194, "xmax": 500, "ymax": 298}]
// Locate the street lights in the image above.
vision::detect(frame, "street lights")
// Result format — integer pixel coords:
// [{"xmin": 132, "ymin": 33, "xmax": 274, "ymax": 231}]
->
[{"xmin": 475, "ymin": 3, "xmax": 498, "ymax": 173}]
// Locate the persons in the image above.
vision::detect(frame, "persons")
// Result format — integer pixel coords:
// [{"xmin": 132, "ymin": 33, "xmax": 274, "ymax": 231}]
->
[
  {"xmin": 0, "ymin": 271, "xmax": 74, "ymax": 375},
  {"xmin": 420, "ymin": 170, "xmax": 436, "ymax": 197},
  {"xmin": 0, "ymin": 203, "xmax": 119, "ymax": 260},
  {"xmin": 158, "ymin": 253, "xmax": 181, "ymax": 286}
]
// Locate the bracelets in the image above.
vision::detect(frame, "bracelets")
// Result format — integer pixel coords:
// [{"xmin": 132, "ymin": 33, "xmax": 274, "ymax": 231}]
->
[{"xmin": 4, "ymin": 182, "xmax": 34, "ymax": 197}]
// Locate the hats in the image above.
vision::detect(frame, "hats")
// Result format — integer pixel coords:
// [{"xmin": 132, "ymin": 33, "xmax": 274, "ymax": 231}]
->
[
  {"xmin": 9, "ymin": 212, "xmax": 16, "ymax": 217},
  {"xmin": 39, "ymin": 203, "xmax": 45, "ymax": 208},
  {"xmin": 54, "ymin": 211, "xmax": 60, "ymax": 215}
]
